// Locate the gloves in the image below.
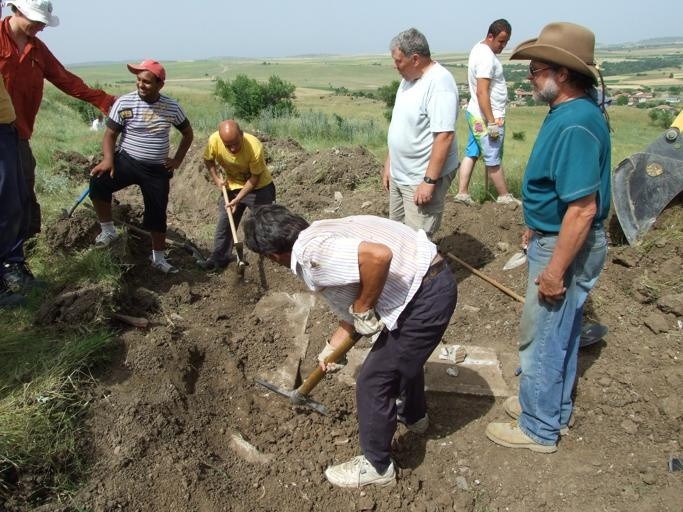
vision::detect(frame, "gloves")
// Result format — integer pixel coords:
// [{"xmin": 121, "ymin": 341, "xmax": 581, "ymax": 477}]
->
[
  {"xmin": 317, "ymin": 338, "xmax": 349, "ymax": 375},
  {"xmin": 349, "ymin": 305, "xmax": 383, "ymax": 339}
]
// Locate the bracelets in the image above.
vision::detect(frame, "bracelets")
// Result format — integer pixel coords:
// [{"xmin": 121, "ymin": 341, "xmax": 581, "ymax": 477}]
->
[{"xmin": 423, "ymin": 176, "xmax": 437, "ymax": 184}]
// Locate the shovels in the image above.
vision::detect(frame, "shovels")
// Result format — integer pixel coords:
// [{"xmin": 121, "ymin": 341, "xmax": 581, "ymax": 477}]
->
[
  {"xmin": 445, "ymin": 248, "xmax": 608, "ymax": 350},
  {"xmin": 81, "ymin": 200, "xmax": 205, "ymax": 262}
]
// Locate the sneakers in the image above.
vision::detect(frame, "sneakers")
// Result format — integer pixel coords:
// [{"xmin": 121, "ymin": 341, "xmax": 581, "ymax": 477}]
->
[
  {"xmin": 90, "ymin": 225, "xmax": 122, "ymax": 251},
  {"xmin": 491, "ymin": 191, "xmax": 522, "ymax": 209},
  {"xmin": 394, "ymin": 413, "xmax": 430, "ymax": 441},
  {"xmin": 2, "ymin": 259, "xmax": 36, "ymax": 293},
  {"xmin": 324, "ymin": 453, "xmax": 400, "ymax": 492},
  {"xmin": 205, "ymin": 258, "xmax": 230, "ymax": 272},
  {"xmin": 485, "ymin": 392, "xmax": 576, "ymax": 453},
  {"xmin": 458, "ymin": 192, "xmax": 475, "ymax": 210},
  {"xmin": 149, "ymin": 257, "xmax": 182, "ymax": 278}
]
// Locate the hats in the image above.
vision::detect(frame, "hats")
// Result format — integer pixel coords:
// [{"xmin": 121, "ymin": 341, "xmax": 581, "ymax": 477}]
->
[
  {"xmin": 6, "ymin": 2, "xmax": 63, "ymax": 30},
  {"xmin": 508, "ymin": 20, "xmax": 605, "ymax": 85},
  {"xmin": 125, "ymin": 59, "xmax": 165, "ymax": 83}
]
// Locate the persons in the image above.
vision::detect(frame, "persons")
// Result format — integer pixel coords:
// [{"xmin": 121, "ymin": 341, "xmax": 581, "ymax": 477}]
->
[
  {"xmin": 88, "ymin": 60, "xmax": 194, "ymax": 276},
  {"xmin": 454, "ymin": 19, "xmax": 523, "ymax": 207},
  {"xmin": 242, "ymin": 203, "xmax": 457, "ymax": 490},
  {"xmin": 196, "ymin": 119, "xmax": 276, "ymax": 274},
  {"xmin": 90, "ymin": 115, "xmax": 106, "ymax": 131},
  {"xmin": 486, "ymin": 22, "xmax": 612, "ymax": 454},
  {"xmin": 0, "ymin": 73, "xmax": 22, "ymax": 260},
  {"xmin": 0, "ymin": 0, "xmax": 120, "ymax": 266},
  {"xmin": 382, "ymin": 26, "xmax": 459, "ymax": 241}
]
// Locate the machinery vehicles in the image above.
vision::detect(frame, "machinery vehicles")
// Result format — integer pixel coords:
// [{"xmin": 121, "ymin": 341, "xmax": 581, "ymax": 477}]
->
[{"xmin": 611, "ymin": 101, "xmax": 682, "ymax": 251}]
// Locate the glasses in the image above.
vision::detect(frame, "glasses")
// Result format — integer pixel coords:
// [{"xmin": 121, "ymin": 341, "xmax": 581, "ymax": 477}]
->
[{"xmin": 529, "ymin": 63, "xmax": 553, "ymax": 76}]
